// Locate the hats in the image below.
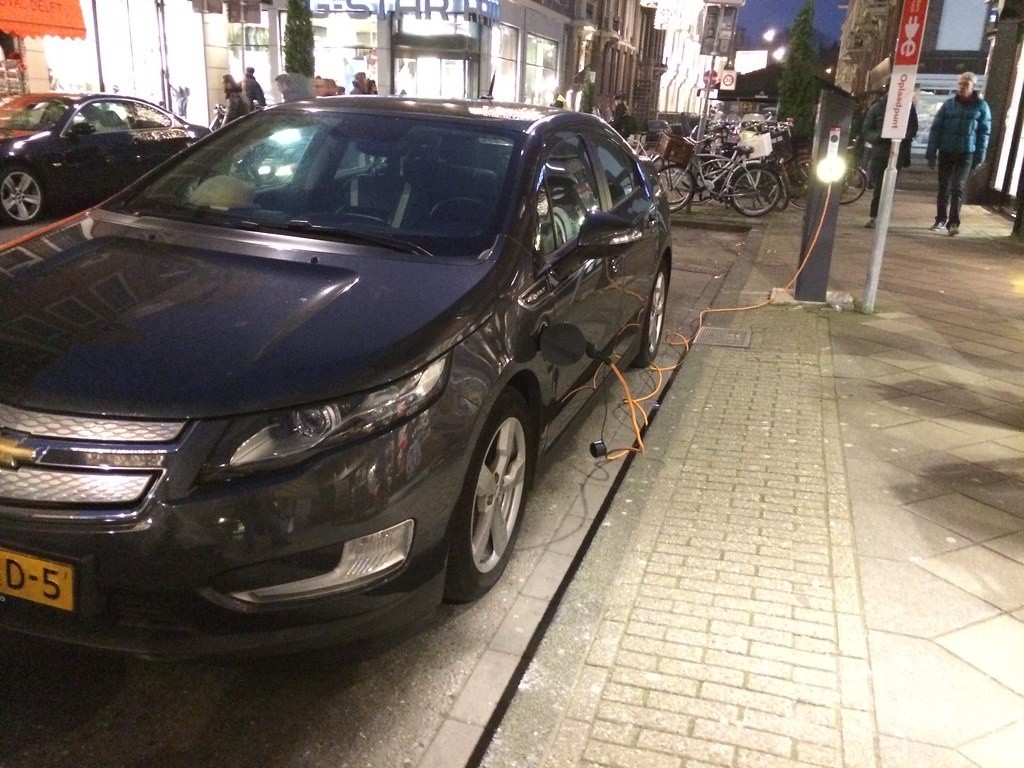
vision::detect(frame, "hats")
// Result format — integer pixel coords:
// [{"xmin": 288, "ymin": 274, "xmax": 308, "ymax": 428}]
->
[{"xmin": 958, "ymin": 72, "xmax": 976, "ymax": 84}]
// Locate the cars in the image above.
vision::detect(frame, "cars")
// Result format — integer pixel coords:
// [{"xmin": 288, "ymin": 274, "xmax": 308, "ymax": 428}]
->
[
  {"xmin": 0, "ymin": 95, "xmax": 675, "ymax": 668},
  {"xmin": 0, "ymin": 90, "xmax": 213, "ymax": 228}
]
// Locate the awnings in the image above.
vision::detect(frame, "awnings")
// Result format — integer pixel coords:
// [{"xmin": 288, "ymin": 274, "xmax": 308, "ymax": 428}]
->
[{"xmin": 0, "ymin": 0, "xmax": 87, "ymax": 42}]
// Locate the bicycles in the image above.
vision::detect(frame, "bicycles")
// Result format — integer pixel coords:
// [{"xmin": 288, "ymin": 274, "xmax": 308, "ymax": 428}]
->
[{"xmin": 654, "ymin": 111, "xmax": 871, "ymax": 217}]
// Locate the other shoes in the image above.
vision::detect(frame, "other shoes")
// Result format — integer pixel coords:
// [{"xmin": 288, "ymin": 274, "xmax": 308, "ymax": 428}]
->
[
  {"xmin": 865, "ymin": 219, "xmax": 875, "ymax": 228},
  {"xmin": 931, "ymin": 220, "xmax": 946, "ymax": 231},
  {"xmin": 948, "ymin": 222, "xmax": 959, "ymax": 235}
]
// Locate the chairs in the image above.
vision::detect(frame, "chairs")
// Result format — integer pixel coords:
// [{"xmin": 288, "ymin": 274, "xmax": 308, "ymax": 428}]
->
[
  {"xmin": 84, "ymin": 105, "xmax": 122, "ymax": 129},
  {"xmin": 330, "ymin": 164, "xmax": 501, "ymax": 220}
]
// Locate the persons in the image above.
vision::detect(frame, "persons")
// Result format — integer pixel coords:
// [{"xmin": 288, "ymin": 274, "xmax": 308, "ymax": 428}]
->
[
  {"xmin": 607, "ymin": 96, "xmax": 635, "ymax": 140},
  {"xmin": 861, "ymin": 87, "xmax": 919, "ymax": 228},
  {"xmin": 351, "ymin": 71, "xmax": 377, "ymax": 95},
  {"xmin": 924, "ymin": 71, "xmax": 993, "ymax": 236},
  {"xmin": 221, "ymin": 65, "xmax": 346, "ymax": 186}
]
